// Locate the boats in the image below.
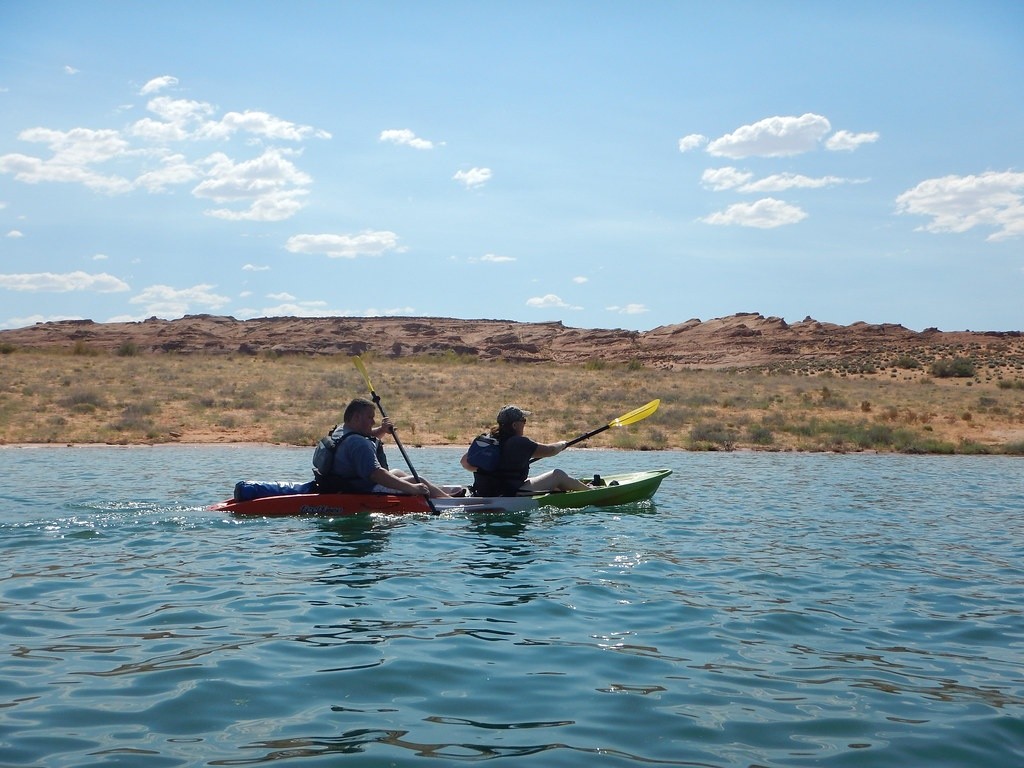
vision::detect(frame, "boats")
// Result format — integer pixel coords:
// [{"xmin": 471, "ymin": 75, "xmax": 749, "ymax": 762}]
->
[{"xmin": 204, "ymin": 468, "xmax": 676, "ymax": 518}]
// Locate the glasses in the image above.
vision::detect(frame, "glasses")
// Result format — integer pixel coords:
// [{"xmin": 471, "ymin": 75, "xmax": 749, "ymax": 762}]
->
[{"xmin": 516, "ymin": 419, "xmax": 526, "ymax": 423}]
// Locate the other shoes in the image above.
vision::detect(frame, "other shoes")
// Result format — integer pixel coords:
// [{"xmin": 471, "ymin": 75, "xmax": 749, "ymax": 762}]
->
[
  {"xmin": 608, "ymin": 480, "xmax": 619, "ymax": 486},
  {"xmin": 449, "ymin": 489, "xmax": 466, "ymax": 497},
  {"xmin": 591, "ymin": 474, "xmax": 600, "ymax": 486}
]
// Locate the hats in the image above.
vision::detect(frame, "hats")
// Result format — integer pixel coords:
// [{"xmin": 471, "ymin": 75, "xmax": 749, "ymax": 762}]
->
[{"xmin": 497, "ymin": 406, "xmax": 532, "ymax": 423}]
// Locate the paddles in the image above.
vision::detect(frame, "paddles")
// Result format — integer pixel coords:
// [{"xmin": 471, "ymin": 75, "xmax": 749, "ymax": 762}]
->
[
  {"xmin": 529, "ymin": 397, "xmax": 662, "ymax": 466},
  {"xmin": 350, "ymin": 353, "xmax": 440, "ymax": 515}
]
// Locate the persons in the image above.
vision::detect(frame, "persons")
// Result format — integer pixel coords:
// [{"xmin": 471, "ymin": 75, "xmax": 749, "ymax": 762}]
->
[
  {"xmin": 459, "ymin": 405, "xmax": 620, "ymax": 496},
  {"xmin": 312, "ymin": 397, "xmax": 467, "ymax": 498}
]
